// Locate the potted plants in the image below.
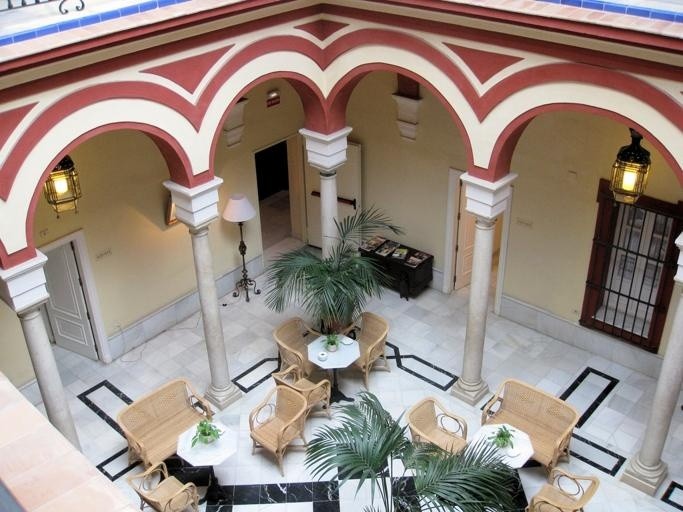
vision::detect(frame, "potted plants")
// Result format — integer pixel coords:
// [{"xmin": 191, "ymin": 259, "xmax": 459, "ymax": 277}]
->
[
  {"xmin": 320, "ymin": 332, "xmax": 339, "ymax": 352},
  {"xmin": 190, "ymin": 418, "xmax": 221, "ymax": 448},
  {"xmin": 487, "ymin": 425, "xmax": 515, "ymax": 449}
]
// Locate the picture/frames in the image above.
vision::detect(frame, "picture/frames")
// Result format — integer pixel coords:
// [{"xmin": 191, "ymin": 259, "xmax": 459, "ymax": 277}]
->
[{"xmin": 167, "ymin": 193, "xmax": 178, "ymax": 225}]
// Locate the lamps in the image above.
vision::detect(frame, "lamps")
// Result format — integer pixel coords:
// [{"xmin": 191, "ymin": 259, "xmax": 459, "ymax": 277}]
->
[
  {"xmin": 221, "ymin": 193, "xmax": 261, "ymax": 302},
  {"xmin": 43, "ymin": 154, "xmax": 82, "ymax": 218},
  {"xmin": 608, "ymin": 127, "xmax": 651, "ymax": 204}
]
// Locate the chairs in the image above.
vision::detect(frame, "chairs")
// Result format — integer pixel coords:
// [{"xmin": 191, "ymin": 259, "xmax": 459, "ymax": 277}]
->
[
  {"xmin": 272, "ymin": 317, "xmax": 325, "ymax": 384},
  {"xmin": 406, "ymin": 396, "xmax": 467, "ymax": 467},
  {"xmin": 271, "ymin": 364, "xmax": 332, "ymax": 420},
  {"xmin": 524, "ymin": 468, "xmax": 600, "ymax": 512},
  {"xmin": 338, "ymin": 312, "xmax": 391, "ymax": 392},
  {"xmin": 126, "ymin": 461, "xmax": 201, "ymax": 512},
  {"xmin": 248, "ymin": 384, "xmax": 309, "ymax": 477}
]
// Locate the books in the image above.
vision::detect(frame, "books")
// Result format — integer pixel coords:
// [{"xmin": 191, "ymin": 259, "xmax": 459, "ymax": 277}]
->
[{"xmin": 361, "ymin": 235, "xmax": 431, "ymax": 269}]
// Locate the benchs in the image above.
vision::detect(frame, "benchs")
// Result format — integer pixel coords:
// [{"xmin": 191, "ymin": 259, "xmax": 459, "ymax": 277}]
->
[
  {"xmin": 116, "ymin": 378, "xmax": 212, "ymax": 479},
  {"xmin": 481, "ymin": 378, "xmax": 580, "ymax": 478}
]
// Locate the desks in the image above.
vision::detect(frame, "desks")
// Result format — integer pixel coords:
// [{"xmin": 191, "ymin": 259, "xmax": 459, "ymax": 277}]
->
[
  {"xmin": 472, "ymin": 424, "xmax": 534, "ymax": 470},
  {"xmin": 177, "ymin": 421, "xmax": 236, "ymax": 506},
  {"xmin": 307, "ymin": 334, "xmax": 360, "ymax": 409},
  {"xmin": 359, "ymin": 237, "xmax": 434, "ymax": 301}
]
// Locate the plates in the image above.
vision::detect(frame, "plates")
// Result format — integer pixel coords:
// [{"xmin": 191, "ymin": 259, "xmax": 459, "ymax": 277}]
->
[
  {"xmin": 340, "ymin": 336, "xmax": 353, "ymax": 345},
  {"xmin": 506, "ymin": 449, "xmax": 520, "ymax": 457}
]
